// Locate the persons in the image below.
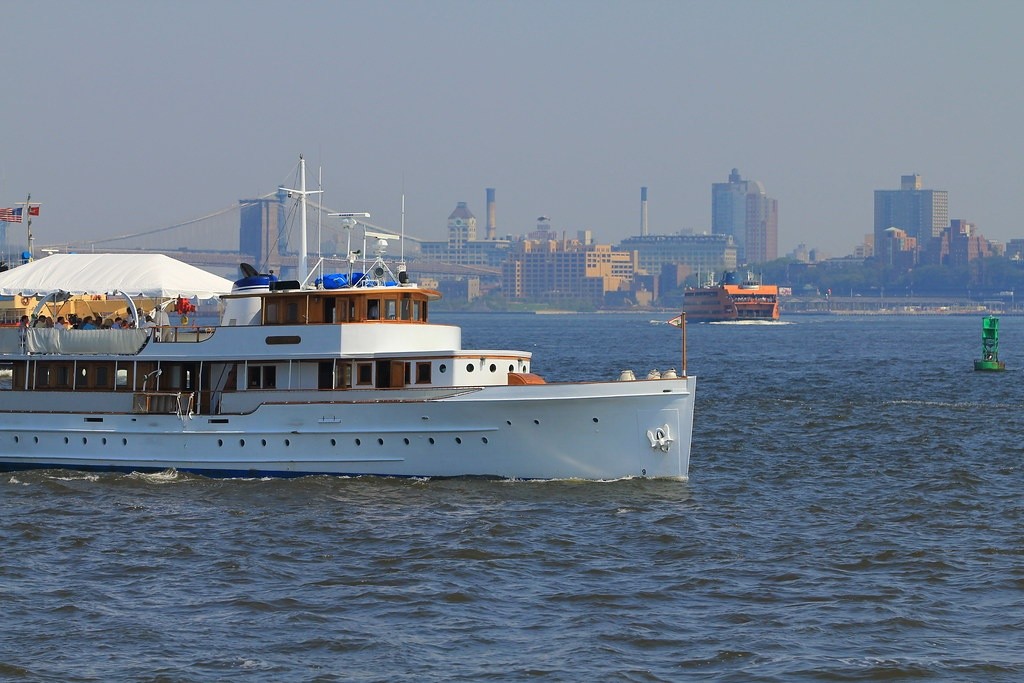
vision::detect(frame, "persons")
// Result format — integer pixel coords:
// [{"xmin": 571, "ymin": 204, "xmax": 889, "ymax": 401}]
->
[{"xmin": 19, "ymin": 305, "xmax": 172, "ymax": 355}]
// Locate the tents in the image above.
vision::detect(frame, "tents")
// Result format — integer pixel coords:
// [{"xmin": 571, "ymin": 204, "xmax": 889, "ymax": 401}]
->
[{"xmin": 0, "ymin": 253, "xmax": 235, "ymax": 330}]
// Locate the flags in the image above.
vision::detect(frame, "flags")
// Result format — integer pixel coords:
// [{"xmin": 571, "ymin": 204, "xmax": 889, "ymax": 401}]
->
[
  {"xmin": 29, "ymin": 206, "xmax": 39, "ymax": 217},
  {"xmin": 0, "ymin": 207, "xmax": 23, "ymax": 223}
]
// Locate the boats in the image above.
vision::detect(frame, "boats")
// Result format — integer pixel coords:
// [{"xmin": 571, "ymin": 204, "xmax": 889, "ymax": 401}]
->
[
  {"xmin": 681, "ymin": 269, "xmax": 781, "ymax": 325},
  {"xmin": 973, "ymin": 317, "xmax": 1007, "ymax": 372},
  {"xmin": 0, "ymin": 156, "xmax": 699, "ymax": 486}
]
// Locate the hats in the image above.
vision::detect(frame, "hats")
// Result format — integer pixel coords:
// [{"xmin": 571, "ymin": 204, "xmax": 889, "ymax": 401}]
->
[
  {"xmin": 154, "ymin": 305, "xmax": 161, "ymax": 309},
  {"xmin": 39, "ymin": 315, "xmax": 47, "ymax": 319}
]
